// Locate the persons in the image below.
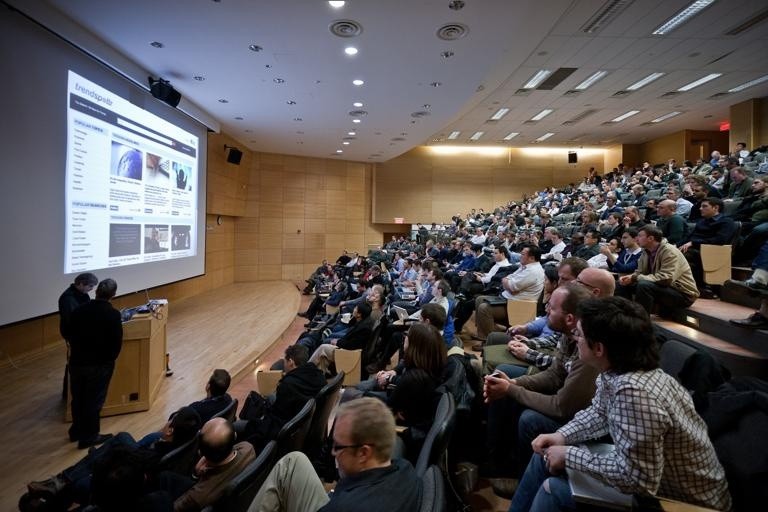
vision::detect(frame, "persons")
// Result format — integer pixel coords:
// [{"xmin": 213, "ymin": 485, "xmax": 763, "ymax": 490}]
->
[
  {"xmin": 18, "ymin": 323, "xmax": 449, "ymax": 512},
  {"xmin": 482, "ymin": 282, "xmax": 732, "ymax": 512},
  {"xmin": 68, "ymin": 278, "xmax": 123, "ymax": 450},
  {"xmin": 151, "ymin": 227, "xmax": 159, "ymax": 247},
  {"xmin": 176, "ymin": 169, "xmax": 187, "ymax": 189},
  {"xmin": 58, "ymin": 272, "xmax": 97, "ymax": 402},
  {"xmin": 296, "ymin": 141, "xmax": 768, "ymax": 381}
]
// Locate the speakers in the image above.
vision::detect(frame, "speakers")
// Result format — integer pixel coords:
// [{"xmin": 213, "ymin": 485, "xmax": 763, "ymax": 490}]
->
[
  {"xmin": 151, "ymin": 83, "xmax": 181, "ymax": 108},
  {"xmin": 227, "ymin": 149, "xmax": 242, "ymax": 165},
  {"xmin": 569, "ymin": 153, "xmax": 576, "ymax": 163}
]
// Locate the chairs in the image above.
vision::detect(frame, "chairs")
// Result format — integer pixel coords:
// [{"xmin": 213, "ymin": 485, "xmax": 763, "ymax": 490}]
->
[
  {"xmin": 256, "ymin": 219, "xmax": 763, "ymax": 393},
  {"xmin": 27, "ymin": 395, "xmax": 766, "ymax": 511}
]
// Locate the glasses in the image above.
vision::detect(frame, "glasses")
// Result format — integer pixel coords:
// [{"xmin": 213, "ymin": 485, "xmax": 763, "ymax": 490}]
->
[
  {"xmin": 573, "ymin": 278, "xmax": 594, "ymax": 289},
  {"xmin": 333, "ymin": 443, "xmax": 363, "ymax": 452}
]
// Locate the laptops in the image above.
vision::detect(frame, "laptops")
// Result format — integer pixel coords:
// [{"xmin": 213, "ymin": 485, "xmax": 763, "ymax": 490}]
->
[
  {"xmin": 483, "ymin": 298, "xmax": 507, "ymax": 304},
  {"xmin": 393, "ymin": 305, "xmax": 410, "ymax": 323},
  {"xmin": 396, "ymin": 280, "xmax": 414, "ymax": 293},
  {"xmin": 394, "ymin": 286, "xmax": 415, "ymax": 300},
  {"xmin": 566, "ymin": 443, "xmax": 636, "ymax": 509}
]
